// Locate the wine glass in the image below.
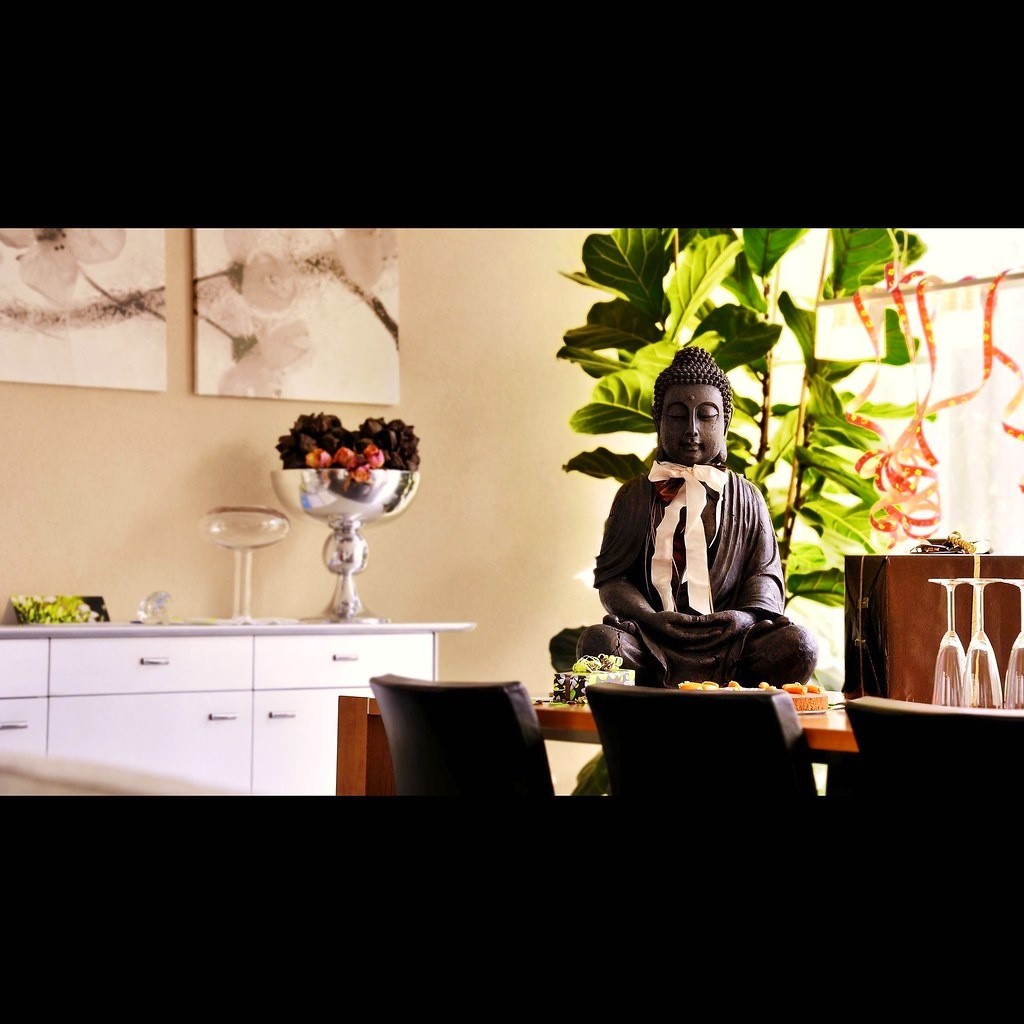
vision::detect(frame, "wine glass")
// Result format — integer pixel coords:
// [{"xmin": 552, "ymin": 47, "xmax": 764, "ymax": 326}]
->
[
  {"xmin": 929, "ymin": 579, "xmax": 970, "ymax": 708},
  {"xmin": 958, "ymin": 578, "xmax": 1003, "ymax": 710},
  {"xmin": 1002, "ymin": 579, "xmax": 1024, "ymax": 710}
]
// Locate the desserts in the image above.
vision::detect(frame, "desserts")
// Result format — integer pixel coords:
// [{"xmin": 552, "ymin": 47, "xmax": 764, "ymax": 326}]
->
[
  {"xmin": 553, "ymin": 669, "xmax": 635, "ymax": 701},
  {"xmin": 679, "ymin": 680, "xmax": 828, "ymax": 711}
]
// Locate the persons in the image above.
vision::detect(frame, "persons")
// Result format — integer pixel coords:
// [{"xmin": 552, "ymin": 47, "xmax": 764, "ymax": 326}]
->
[{"xmin": 576, "ymin": 348, "xmax": 818, "ymax": 691}]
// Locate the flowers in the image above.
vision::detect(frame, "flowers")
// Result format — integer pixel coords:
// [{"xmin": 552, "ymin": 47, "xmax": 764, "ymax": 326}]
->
[{"xmin": 275, "ymin": 412, "xmax": 420, "ymax": 483}]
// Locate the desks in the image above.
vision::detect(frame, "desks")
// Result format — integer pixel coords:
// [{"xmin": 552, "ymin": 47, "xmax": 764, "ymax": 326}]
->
[{"xmin": 337, "ymin": 693, "xmax": 860, "ymax": 798}]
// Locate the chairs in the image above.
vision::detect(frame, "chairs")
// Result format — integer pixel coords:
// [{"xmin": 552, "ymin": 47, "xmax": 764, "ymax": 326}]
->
[
  {"xmin": 586, "ymin": 683, "xmax": 820, "ymax": 797},
  {"xmin": 845, "ymin": 695, "xmax": 1024, "ymax": 798},
  {"xmin": 371, "ymin": 674, "xmax": 555, "ymax": 797}
]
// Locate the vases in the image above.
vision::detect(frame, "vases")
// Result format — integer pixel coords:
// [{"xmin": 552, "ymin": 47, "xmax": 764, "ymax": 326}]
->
[{"xmin": 270, "ymin": 468, "xmax": 419, "ymax": 624}]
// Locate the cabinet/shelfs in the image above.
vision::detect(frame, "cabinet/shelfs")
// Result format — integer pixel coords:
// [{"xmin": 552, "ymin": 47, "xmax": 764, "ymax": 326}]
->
[{"xmin": 1, "ymin": 621, "xmax": 476, "ymax": 796}]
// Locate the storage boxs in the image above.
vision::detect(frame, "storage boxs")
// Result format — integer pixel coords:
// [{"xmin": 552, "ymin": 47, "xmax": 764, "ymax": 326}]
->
[
  {"xmin": 553, "ymin": 668, "xmax": 634, "ymax": 705},
  {"xmin": 843, "ymin": 556, "xmax": 1023, "ymax": 710}
]
